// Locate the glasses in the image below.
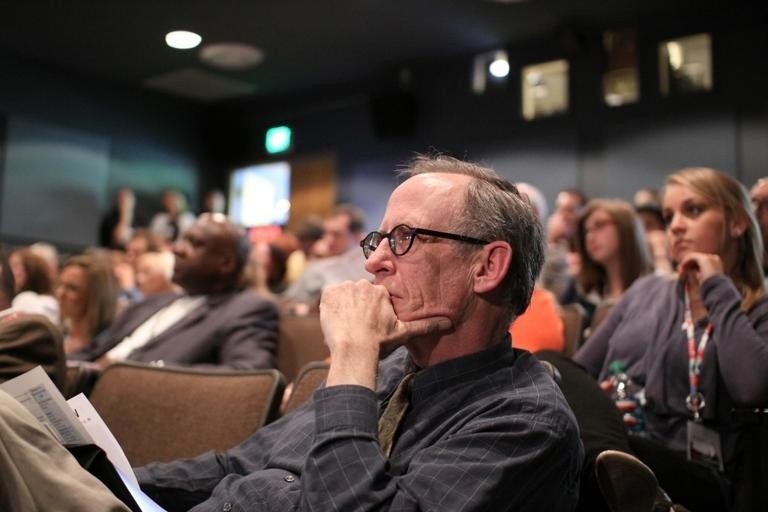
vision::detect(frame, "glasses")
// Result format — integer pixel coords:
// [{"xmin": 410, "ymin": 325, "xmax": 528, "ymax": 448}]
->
[{"xmin": 360, "ymin": 224, "xmax": 491, "ymax": 260}]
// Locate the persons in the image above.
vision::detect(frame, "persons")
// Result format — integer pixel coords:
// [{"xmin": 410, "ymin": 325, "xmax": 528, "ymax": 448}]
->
[
  {"xmin": 1, "ymin": 188, "xmax": 365, "ymax": 377},
  {"xmin": 534, "ymin": 166, "xmax": 768, "ymax": 512},
  {"xmin": 2, "ymin": 157, "xmax": 585, "ymax": 511},
  {"xmin": 515, "ymin": 176, "xmax": 673, "ymax": 349},
  {"xmin": 748, "ymin": 177, "xmax": 768, "ymax": 245}
]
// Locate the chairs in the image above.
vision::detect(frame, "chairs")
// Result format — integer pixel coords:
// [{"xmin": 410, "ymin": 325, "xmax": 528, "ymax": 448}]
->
[
  {"xmin": 89, "ymin": 361, "xmax": 287, "ymax": 468},
  {"xmin": 561, "ymin": 301, "xmax": 589, "ymax": 357},
  {"xmin": 66, "ymin": 366, "xmax": 94, "ymax": 398},
  {"xmin": 593, "ymin": 296, "xmax": 621, "ymax": 330},
  {"xmin": 285, "ymin": 361, "xmax": 330, "ymax": 414},
  {"xmin": 275, "ymin": 315, "xmax": 328, "ymax": 375}
]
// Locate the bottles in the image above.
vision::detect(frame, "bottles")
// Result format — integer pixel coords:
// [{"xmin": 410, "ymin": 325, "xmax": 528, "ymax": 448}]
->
[{"xmin": 608, "ymin": 360, "xmax": 652, "ymax": 440}]
